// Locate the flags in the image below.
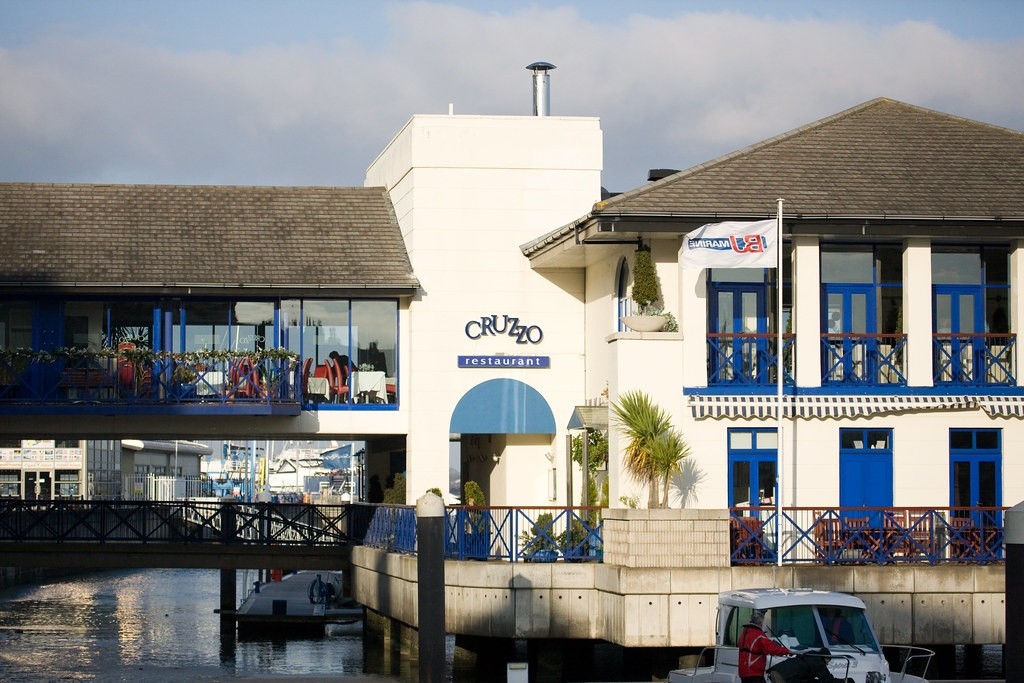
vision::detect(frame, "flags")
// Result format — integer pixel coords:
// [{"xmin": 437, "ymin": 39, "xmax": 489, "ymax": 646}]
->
[{"xmin": 677, "ymin": 219, "xmax": 779, "ymax": 272}]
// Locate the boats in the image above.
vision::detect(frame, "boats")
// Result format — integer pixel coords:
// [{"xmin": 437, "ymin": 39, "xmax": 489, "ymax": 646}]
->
[{"xmin": 668, "ymin": 585, "xmax": 936, "ymax": 683}]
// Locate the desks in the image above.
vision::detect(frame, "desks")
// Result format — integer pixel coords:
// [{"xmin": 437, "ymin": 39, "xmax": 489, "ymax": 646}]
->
[
  {"xmin": 947, "ymin": 526, "xmax": 1002, "ymax": 565},
  {"xmin": 843, "ymin": 527, "xmax": 911, "ymax": 566},
  {"xmin": 344, "ymin": 372, "xmax": 389, "ymax": 404},
  {"xmin": 194, "ymin": 370, "xmax": 231, "ymax": 400},
  {"xmin": 307, "ymin": 379, "xmax": 330, "ymax": 404}
]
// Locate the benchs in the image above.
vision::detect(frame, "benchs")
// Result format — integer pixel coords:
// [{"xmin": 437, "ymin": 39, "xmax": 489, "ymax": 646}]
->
[{"xmin": 54, "ymin": 367, "xmax": 115, "ymax": 401}]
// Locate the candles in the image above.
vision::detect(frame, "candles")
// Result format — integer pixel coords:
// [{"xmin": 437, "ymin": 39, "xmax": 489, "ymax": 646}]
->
[{"xmin": 358, "ymin": 363, "xmax": 375, "ymax": 371}]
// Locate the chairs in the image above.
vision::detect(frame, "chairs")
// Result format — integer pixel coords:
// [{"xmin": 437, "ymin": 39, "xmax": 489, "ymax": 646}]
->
[
  {"xmin": 809, "ymin": 511, "xmax": 870, "ymax": 566},
  {"xmin": 226, "ymin": 357, "xmax": 263, "ymax": 402},
  {"xmin": 300, "ymin": 357, "xmax": 314, "ymax": 396},
  {"xmin": 884, "ymin": 516, "xmax": 938, "ymax": 563},
  {"xmin": 950, "ymin": 517, "xmax": 974, "ymax": 558},
  {"xmin": 386, "ymin": 381, "xmax": 396, "ymax": 396},
  {"xmin": 315, "ymin": 362, "xmax": 349, "ymax": 405},
  {"xmin": 730, "ymin": 517, "xmax": 767, "ymax": 566}
]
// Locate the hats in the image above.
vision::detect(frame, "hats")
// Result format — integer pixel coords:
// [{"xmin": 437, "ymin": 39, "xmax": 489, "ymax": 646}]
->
[{"xmin": 820, "ymin": 647, "xmax": 831, "ymax": 660}]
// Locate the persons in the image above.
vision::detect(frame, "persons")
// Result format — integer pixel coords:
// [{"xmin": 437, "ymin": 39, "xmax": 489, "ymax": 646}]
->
[
  {"xmin": 737, "ymin": 613, "xmax": 791, "ymax": 683},
  {"xmin": 329, "ymin": 351, "xmax": 356, "ymax": 403}
]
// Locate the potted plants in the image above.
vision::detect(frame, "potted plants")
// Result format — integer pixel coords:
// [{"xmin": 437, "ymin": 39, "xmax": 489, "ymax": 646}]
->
[
  {"xmin": 595, "ymin": 474, "xmax": 609, "ymax": 562},
  {"xmin": 258, "ymin": 363, "xmax": 280, "ymax": 402},
  {"xmin": 618, "ymin": 249, "xmax": 677, "ymax": 332},
  {"xmin": 894, "ymin": 305, "xmax": 903, "ymax": 381},
  {"xmin": 784, "ymin": 312, "xmax": 792, "ymax": 383},
  {"xmin": 558, "ymin": 518, "xmax": 589, "ymax": 563},
  {"xmin": 518, "ymin": 512, "xmax": 560, "ymax": 562},
  {"xmin": 171, "ymin": 366, "xmax": 197, "ymax": 401}
]
